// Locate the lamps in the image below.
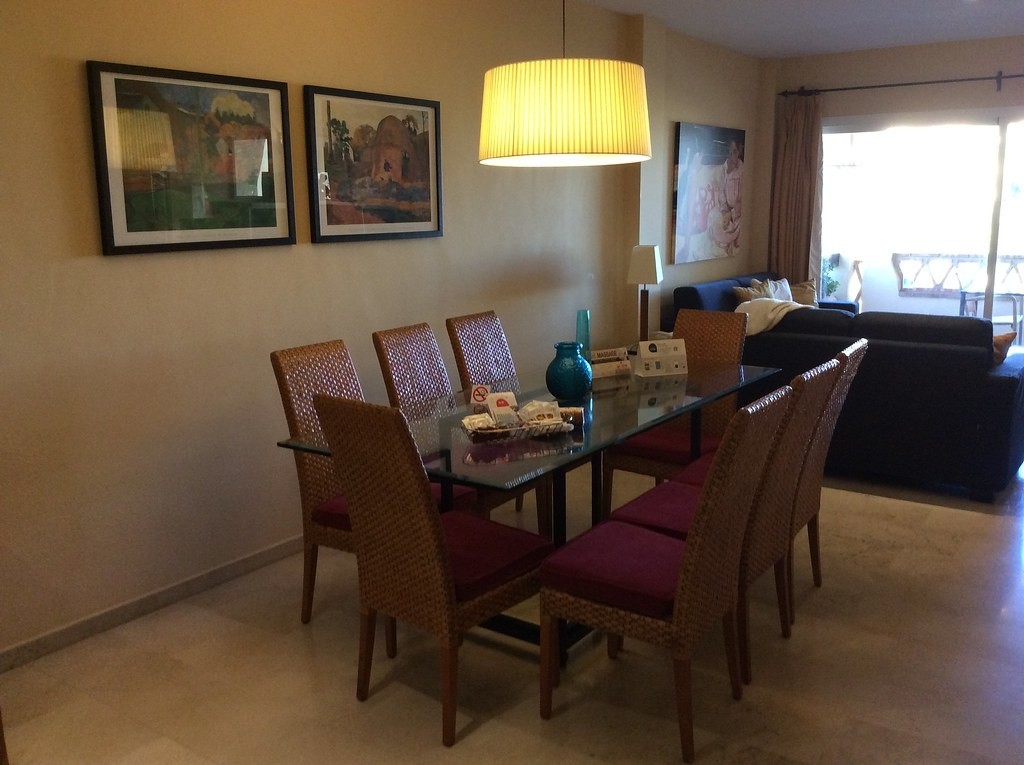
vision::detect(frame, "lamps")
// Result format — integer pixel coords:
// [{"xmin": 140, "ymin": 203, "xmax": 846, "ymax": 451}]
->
[
  {"xmin": 478, "ymin": 0, "xmax": 653, "ymax": 168},
  {"xmin": 626, "ymin": 245, "xmax": 664, "ymax": 345}
]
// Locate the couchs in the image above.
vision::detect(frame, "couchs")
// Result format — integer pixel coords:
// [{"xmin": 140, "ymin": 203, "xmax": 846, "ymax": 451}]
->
[{"xmin": 661, "ymin": 272, "xmax": 1024, "ymax": 505}]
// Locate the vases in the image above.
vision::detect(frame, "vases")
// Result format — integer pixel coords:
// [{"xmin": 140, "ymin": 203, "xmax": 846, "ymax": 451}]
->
[{"xmin": 545, "ymin": 341, "xmax": 594, "ymax": 402}]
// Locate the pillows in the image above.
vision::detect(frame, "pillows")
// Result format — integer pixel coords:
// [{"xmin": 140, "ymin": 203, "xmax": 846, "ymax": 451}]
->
[
  {"xmin": 790, "ymin": 278, "xmax": 819, "ymax": 308},
  {"xmin": 994, "ymin": 332, "xmax": 1018, "ymax": 367},
  {"xmin": 752, "ymin": 277, "xmax": 793, "ymax": 302},
  {"xmin": 732, "ymin": 278, "xmax": 776, "ymax": 306}
]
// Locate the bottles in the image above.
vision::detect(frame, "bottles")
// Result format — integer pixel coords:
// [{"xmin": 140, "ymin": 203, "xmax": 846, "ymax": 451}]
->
[{"xmin": 545, "ymin": 339, "xmax": 593, "ymax": 404}]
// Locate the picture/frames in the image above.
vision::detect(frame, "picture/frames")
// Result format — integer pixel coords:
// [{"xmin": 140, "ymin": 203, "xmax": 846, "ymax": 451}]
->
[
  {"xmin": 303, "ymin": 84, "xmax": 443, "ymax": 243},
  {"xmin": 86, "ymin": 58, "xmax": 296, "ymax": 258}
]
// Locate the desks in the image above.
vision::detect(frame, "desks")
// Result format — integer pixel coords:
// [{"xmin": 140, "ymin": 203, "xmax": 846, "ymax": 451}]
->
[{"xmin": 276, "ymin": 356, "xmax": 780, "ymax": 689}]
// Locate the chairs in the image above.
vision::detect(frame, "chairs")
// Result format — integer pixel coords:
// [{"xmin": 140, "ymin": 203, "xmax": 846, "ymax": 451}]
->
[
  {"xmin": 609, "ymin": 357, "xmax": 840, "ymax": 685},
  {"xmin": 371, "ymin": 322, "xmax": 543, "ymax": 534},
  {"xmin": 311, "ymin": 392, "xmax": 545, "ymax": 747},
  {"xmin": 447, "ymin": 309, "xmax": 604, "ymax": 546},
  {"xmin": 603, "ymin": 310, "xmax": 746, "ymax": 520},
  {"xmin": 538, "ymin": 385, "xmax": 796, "ymax": 765},
  {"xmin": 270, "ymin": 339, "xmax": 478, "ymax": 659},
  {"xmin": 670, "ymin": 337, "xmax": 871, "ymax": 625}
]
